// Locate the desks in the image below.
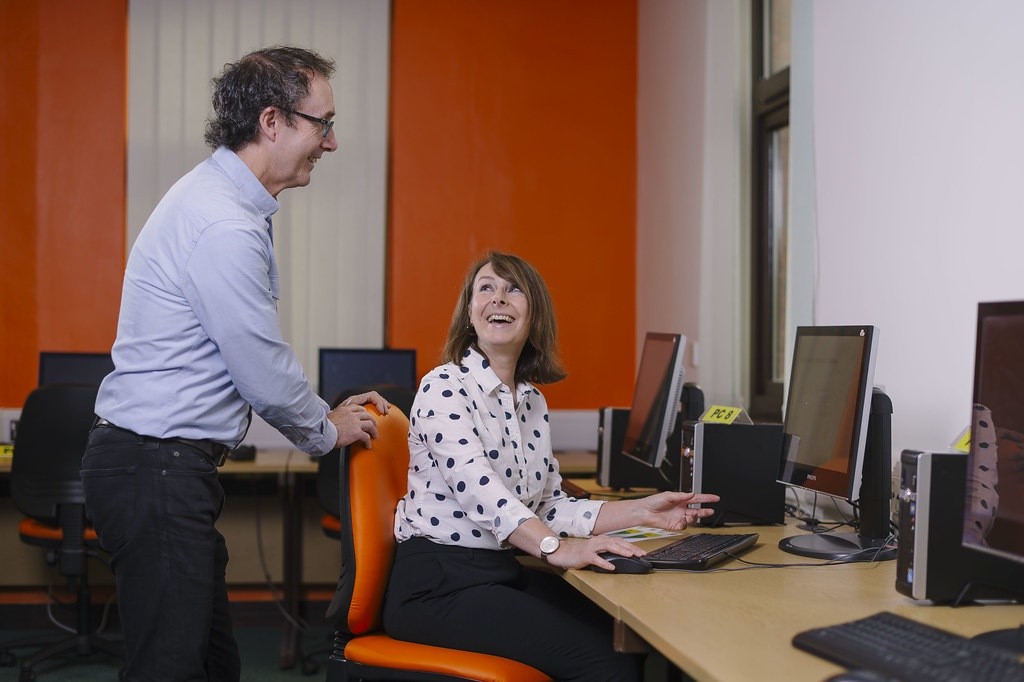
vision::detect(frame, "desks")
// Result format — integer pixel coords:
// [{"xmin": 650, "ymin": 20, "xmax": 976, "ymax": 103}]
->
[
  {"xmin": 545, "ymin": 478, "xmax": 1024, "ymax": 681},
  {"xmin": 0, "ymin": 444, "xmax": 641, "ymax": 668}
]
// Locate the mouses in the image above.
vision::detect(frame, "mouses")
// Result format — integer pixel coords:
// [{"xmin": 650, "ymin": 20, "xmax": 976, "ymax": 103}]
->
[
  {"xmin": 821, "ymin": 670, "xmax": 898, "ymax": 682},
  {"xmin": 590, "ymin": 552, "xmax": 654, "ymax": 574},
  {"xmin": 227, "ymin": 445, "xmax": 258, "ymax": 462}
]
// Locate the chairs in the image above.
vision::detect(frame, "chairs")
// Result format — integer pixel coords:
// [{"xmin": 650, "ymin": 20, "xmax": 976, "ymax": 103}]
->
[{"xmin": 11, "ymin": 383, "xmax": 551, "ymax": 682}]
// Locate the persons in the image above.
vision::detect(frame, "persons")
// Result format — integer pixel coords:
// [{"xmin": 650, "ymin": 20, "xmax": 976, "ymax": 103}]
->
[
  {"xmin": 963, "ymin": 403, "xmax": 1000, "ymax": 550},
  {"xmin": 82, "ymin": 47, "xmax": 392, "ymax": 682},
  {"xmin": 379, "ymin": 251, "xmax": 720, "ymax": 682}
]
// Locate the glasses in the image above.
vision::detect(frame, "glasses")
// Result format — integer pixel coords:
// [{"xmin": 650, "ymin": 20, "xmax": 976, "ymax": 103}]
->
[{"xmin": 265, "ymin": 104, "xmax": 334, "ymax": 138}]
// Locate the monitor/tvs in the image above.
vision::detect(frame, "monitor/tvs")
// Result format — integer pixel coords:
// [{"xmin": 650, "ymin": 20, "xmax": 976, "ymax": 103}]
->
[
  {"xmin": 618, "ymin": 332, "xmax": 704, "ymax": 500},
  {"xmin": 319, "ymin": 349, "xmax": 417, "ymax": 421},
  {"xmin": 38, "ymin": 351, "xmax": 115, "ymax": 388},
  {"xmin": 958, "ymin": 301, "xmax": 1024, "ymax": 657},
  {"xmin": 775, "ymin": 324, "xmax": 898, "ymax": 563}
]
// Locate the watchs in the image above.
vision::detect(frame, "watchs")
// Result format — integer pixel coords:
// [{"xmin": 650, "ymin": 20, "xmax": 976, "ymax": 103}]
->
[{"xmin": 539, "ymin": 535, "xmax": 564, "ymax": 562}]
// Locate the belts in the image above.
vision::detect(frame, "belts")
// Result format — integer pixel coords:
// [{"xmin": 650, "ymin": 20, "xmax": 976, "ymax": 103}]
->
[{"xmin": 96, "ymin": 419, "xmax": 229, "ymax": 467}]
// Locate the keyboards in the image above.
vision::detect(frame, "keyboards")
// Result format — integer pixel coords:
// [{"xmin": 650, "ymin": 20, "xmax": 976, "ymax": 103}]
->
[
  {"xmin": 641, "ymin": 533, "xmax": 760, "ymax": 571},
  {"xmin": 790, "ymin": 611, "xmax": 1024, "ymax": 682}
]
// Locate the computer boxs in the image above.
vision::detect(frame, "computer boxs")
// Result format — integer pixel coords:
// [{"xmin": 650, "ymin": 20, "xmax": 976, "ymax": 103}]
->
[
  {"xmin": 596, "ymin": 407, "xmax": 667, "ymax": 488},
  {"xmin": 678, "ymin": 421, "xmax": 784, "ymax": 524},
  {"xmin": 894, "ymin": 448, "xmax": 1022, "ymax": 606}
]
